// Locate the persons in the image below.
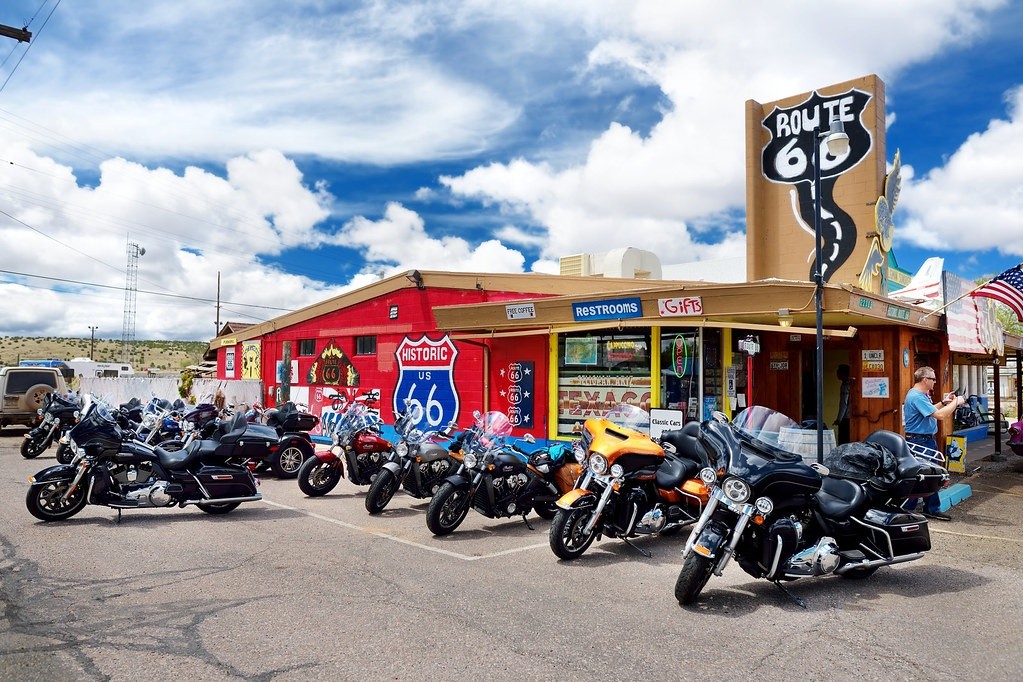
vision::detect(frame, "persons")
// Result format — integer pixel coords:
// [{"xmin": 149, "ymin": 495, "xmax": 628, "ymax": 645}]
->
[
  {"xmin": 902, "ymin": 367, "xmax": 965, "ymax": 521},
  {"xmin": 832, "ymin": 363, "xmax": 851, "ymax": 446}
]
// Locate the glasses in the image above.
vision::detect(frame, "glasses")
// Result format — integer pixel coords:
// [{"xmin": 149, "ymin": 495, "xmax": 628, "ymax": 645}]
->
[{"xmin": 920, "ymin": 377, "xmax": 937, "ymax": 381}]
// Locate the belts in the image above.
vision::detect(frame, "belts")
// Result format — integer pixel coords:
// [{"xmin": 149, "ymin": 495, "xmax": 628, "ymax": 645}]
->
[{"xmin": 905, "ymin": 434, "xmax": 934, "ymax": 438}]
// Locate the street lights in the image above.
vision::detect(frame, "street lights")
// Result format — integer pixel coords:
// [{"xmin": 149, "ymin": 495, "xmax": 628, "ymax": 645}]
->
[{"xmin": 777, "ymin": 119, "xmax": 850, "ymax": 467}]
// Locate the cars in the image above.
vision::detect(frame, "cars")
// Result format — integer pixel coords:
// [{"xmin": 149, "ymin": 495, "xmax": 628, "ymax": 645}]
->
[{"xmin": 1006, "ymin": 414, "xmax": 1023, "ymax": 456}]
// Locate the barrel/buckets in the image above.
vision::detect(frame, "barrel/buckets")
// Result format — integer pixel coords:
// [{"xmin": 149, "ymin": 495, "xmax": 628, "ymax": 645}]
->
[{"xmin": 777, "ymin": 426, "xmax": 837, "ymax": 466}]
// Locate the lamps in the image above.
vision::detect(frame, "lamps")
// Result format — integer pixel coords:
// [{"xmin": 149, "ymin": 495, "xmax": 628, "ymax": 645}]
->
[
  {"xmin": 405, "ymin": 269, "xmax": 424, "ymax": 289},
  {"xmin": 778, "ymin": 316, "xmax": 794, "ymax": 327}
]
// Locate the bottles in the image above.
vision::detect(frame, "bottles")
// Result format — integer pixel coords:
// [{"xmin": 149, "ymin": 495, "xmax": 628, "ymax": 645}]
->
[{"xmin": 578, "ymin": 375, "xmax": 633, "ymax": 386}]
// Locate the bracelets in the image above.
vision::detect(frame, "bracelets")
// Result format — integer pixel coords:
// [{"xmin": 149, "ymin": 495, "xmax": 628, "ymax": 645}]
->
[{"xmin": 941, "ymin": 400, "xmax": 946, "ymax": 406}]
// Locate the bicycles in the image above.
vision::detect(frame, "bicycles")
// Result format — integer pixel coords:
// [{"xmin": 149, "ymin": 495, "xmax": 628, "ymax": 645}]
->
[{"xmin": 953, "ymin": 407, "xmax": 977, "ymax": 431}]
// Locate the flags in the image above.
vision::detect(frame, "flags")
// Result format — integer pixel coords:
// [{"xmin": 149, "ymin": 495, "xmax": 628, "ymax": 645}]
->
[{"xmin": 971, "ymin": 263, "xmax": 1023, "ymax": 322}]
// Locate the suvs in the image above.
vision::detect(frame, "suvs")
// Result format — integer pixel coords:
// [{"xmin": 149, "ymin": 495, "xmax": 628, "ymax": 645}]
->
[{"xmin": 0, "ymin": 367, "xmax": 68, "ymax": 429}]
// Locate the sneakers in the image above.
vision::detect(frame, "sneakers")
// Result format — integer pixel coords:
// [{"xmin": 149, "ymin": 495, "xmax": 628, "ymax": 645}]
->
[{"xmin": 920, "ymin": 505, "xmax": 952, "ymax": 521}]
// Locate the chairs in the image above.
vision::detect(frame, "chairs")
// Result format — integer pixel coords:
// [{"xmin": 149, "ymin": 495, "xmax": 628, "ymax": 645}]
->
[{"xmin": 976, "ymin": 405, "xmax": 1009, "ymax": 434}]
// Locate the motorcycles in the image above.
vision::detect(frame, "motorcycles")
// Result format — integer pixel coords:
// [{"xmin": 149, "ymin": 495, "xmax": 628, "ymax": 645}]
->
[
  {"xmin": 364, "ymin": 401, "xmax": 464, "ymax": 515},
  {"xmin": 24, "ymin": 400, "xmax": 282, "ymax": 524},
  {"xmin": 297, "ymin": 399, "xmax": 397, "ymax": 497},
  {"xmin": 672, "ymin": 406, "xmax": 948, "ymax": 607},
  {"xmin": 425, "ymin": 410, "xmax": 576, "ymax": 538},
  {"xmin": 549, "ymin": 404, "xmax": 710, "ymax": 561},
  {"xmin": 21, "ymin": 386, "xmax": 319, "ymax": 476}
]
